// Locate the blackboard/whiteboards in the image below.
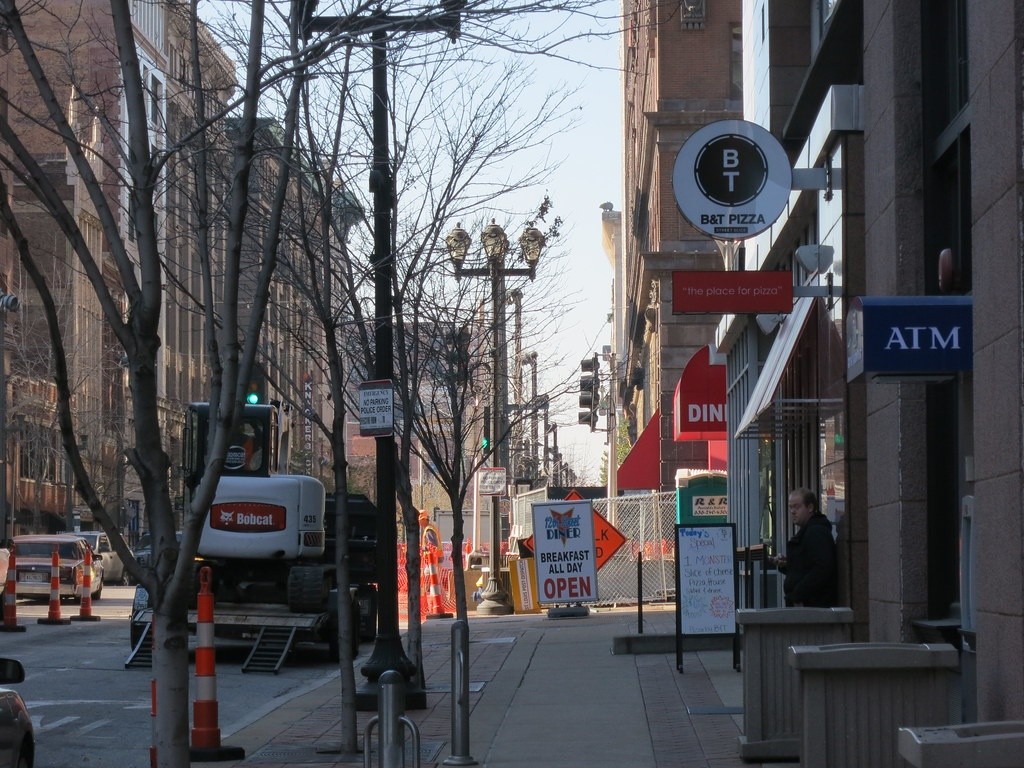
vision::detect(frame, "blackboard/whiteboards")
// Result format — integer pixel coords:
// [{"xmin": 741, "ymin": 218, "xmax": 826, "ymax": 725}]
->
[{"xmin": 674, "ymin": 523, "xmax": 740, "ymax": 639}]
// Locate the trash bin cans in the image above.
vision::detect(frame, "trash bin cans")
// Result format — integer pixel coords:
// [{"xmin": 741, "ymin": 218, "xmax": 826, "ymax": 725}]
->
[
  {"xmin": 898, "ymin": 718, "xmax": 1024, "ymax": 768},
  {"xmin": 734, "ymin": 607, "xmax": 854, "ymax": 765},
  {"xmin": 788, "ymin": 642, "xmax": 959, "ymax": 768}
]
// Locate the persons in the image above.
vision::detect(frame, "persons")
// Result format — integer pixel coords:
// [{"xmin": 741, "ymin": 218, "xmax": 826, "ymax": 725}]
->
[
  {"xmin": 773, "ymin": 487, "xmax": 846, "ymax": 608},
  {"xmin": 229, "ymin": 422, "xmax": 254, "ymax": 474},
  {"xmin": 421, "ymin": 509, "xmax": 445, "ymax": 593}
]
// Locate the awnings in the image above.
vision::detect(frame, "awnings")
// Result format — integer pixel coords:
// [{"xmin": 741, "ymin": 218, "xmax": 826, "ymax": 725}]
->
[
  {"xmin": 672, "ymin": 347, "xmax": 730, "ymax": 443},
  {"xmin": 618, "ymin": 409, "xmax": 660, "ymax": 490}
]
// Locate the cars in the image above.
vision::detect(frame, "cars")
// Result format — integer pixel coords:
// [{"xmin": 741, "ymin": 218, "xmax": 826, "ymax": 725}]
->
[
  {"xmin": 67, "ymin": 531, "xmax": 133, "ymax": 583},
  {"xmin": 0, "ymin": 658, "xmax": 37, "ymax": 768},
  {"xmin": 10, "ymin": 533, "xmax": 104, "ymax": 604},
  {"xmin": 122, "ymin": 529, "xmax": 183, "ymax": 586}
]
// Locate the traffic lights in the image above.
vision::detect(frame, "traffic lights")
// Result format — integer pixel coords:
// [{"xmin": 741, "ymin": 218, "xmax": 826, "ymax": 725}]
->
[
  {"xmin": 482, "ymin": 406, "xmax": 490, "ymax": 455},
  {"xmin": 244, "ymin": 361, "xmax": 264, "ymax": 404},
  {"xmin": 579, "ymin": 359, "xmax": 593, "ymax": 426}
]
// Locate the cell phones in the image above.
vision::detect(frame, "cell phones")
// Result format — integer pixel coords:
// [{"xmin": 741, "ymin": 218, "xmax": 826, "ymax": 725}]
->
[{"xmin": 768, "ymin": 555, "xmax": 785, "ymax": 563}]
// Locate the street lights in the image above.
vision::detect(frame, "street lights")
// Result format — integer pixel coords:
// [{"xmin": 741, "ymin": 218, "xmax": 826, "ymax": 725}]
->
[{"xmin": 444, "ymin": 216, "xmax": 547, "ymax": 614}]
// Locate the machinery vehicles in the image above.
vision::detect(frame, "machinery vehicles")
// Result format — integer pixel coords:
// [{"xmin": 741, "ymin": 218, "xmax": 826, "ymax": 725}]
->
[{"xmin": 123, "ymin": 401, "xmax": 379, "ymax": 675}]
